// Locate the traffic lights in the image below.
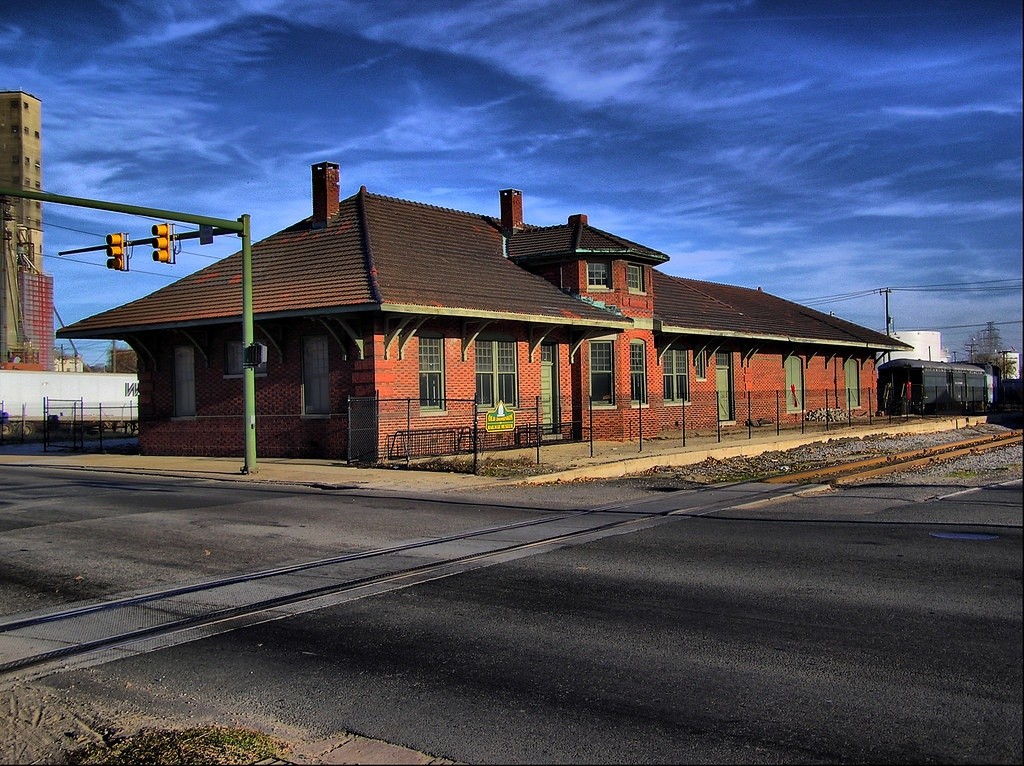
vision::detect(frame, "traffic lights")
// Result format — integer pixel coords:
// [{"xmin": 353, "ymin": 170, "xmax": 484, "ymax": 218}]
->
[
  {"xmin": 150, "ymin": 223, "xmax": 171, "ymax": 264},
  {"xmin": 105, "ymin": 232, "xmax": 125, "ymax": 271}
]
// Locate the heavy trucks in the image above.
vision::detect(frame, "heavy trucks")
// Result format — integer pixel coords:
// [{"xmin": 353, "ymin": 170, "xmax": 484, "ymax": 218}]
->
[{"xmin": 0, "ymin": 369, "xmax": 140, "ymax": 442}]
